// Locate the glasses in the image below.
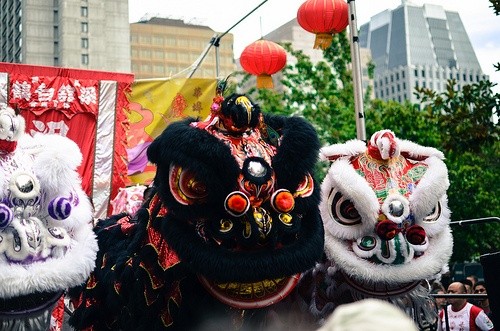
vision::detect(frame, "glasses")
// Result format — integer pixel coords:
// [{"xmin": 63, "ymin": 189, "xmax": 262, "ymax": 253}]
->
[{"xmin": 475, "ymin": 289, "xmax": 485, "ymax": 294}]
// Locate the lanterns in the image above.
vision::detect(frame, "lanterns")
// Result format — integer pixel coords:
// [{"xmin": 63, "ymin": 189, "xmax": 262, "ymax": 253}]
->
[
  {"xmin": 239, "ymin": 37, "xmax": 286, "ymax": 88},
  {"xmin": 297, "ymin": 0, "xmax": 350, "ymax": 49}
]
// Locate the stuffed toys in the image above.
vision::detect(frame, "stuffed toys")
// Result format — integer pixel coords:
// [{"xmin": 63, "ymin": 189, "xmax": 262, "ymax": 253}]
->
[
  {"xmin": 314, "ymin": 130, "xmax": 454, "ymax": 331},
  {"xmin": 66, "ymin": 94, "xmax": 325, "ymax": 331},
  {"xmin": 0, "ymin": 102, "xmax": 99, "ymax": 331}
]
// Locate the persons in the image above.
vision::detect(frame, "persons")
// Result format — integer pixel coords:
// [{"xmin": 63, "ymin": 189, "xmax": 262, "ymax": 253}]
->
[{"xmin": 434, "ymin": 275, "xmax": 496, "ymax": 331}]
